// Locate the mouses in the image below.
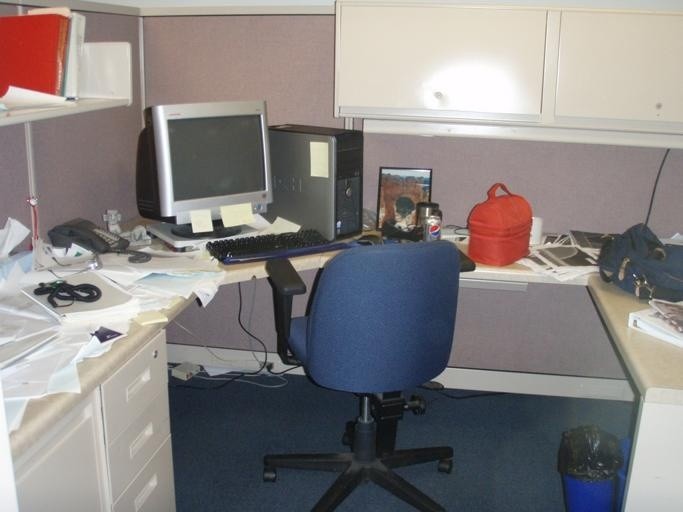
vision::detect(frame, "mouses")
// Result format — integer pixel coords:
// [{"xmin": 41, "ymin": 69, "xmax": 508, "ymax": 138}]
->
[
  {"xmin": 206, "ymin": 229, "xmax": 332, "ymax": 265},
  {"xmin": 357, "ymin": 234, "xmax": 384, "ymax": 246}
]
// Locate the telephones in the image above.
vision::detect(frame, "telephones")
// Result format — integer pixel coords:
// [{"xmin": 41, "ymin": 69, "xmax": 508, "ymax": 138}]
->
[{"xmin": 48, "ymin": 217, "xmax": 129, "ymax": 255}]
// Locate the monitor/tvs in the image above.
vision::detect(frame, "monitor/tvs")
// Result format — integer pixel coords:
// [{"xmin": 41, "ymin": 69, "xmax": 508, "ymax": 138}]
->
[{"xmin": 135, "ymin": 99, "xmax": 274, "ymax": 240}]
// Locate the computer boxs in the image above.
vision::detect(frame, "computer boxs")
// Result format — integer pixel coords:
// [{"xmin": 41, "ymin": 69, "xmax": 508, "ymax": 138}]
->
[{"xmin": 256, "ymin": 123, "xmax": 364, "ymax": 242}]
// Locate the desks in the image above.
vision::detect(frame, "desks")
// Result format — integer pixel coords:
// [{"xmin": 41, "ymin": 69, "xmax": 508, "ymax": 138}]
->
[
  {"xmin": 587, "ymin": 273, "xmax": 683, "ymax": 511},
  {"xmin": 3, "ymin": 215, "xmax": 683, "ymax": 325}
]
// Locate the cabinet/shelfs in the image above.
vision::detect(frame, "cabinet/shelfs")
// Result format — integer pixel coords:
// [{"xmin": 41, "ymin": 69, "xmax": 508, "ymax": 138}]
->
[
  {"xmin": 332, "ymin": 2, "xmax": 682, "ymax": 135},
  {"xmin": 0, "ymin": 327, "xmax": 175, "ymax": 512}
]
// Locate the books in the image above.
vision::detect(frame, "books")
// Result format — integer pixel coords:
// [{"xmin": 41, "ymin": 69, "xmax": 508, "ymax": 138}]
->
[
  {"xmin": 518, "ymin": 228, "xmax": 623, "ymax": 284},
  {"xmin": 0, "ymin": 212, "xmax": 225, "ymax": 440},
  {"xmin": 629, "ymin": 296, "xmax": 682, "ymax": 351}
]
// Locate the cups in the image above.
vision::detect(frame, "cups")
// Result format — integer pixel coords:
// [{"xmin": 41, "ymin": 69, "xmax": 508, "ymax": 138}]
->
[
  {"xmin": 529, "ymin": 216, "xmax": 543, "ymax": 246},
  {"xmin": 416, "ymin": 200, "xmax": 443, "ymax": 243}
]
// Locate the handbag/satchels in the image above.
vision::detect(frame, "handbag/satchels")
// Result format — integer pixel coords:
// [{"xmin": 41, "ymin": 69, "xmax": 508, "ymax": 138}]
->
[
  {"xmin": 466, "ymin": 182, "xmax": 532, "ymax": 268},
  {"xmin": 597, "ymin": 222, "xmax": 683, "ymax": 303}
]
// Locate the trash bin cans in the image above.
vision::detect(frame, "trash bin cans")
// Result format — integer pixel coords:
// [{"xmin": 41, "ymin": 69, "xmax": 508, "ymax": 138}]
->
[{"xmin": 558, "ymin": 427, "xmax": 622, "ymax": 512}]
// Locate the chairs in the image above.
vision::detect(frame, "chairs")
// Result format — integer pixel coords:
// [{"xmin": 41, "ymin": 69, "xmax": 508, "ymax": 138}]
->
[{"xmin": 264, "ymin": 239, "xmax": 458, "ymax": 511}]
